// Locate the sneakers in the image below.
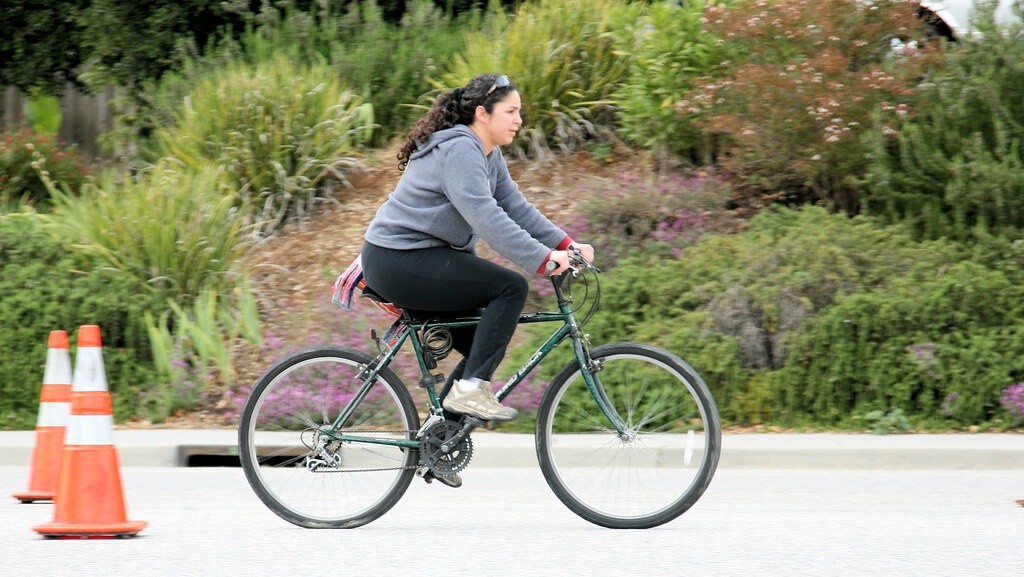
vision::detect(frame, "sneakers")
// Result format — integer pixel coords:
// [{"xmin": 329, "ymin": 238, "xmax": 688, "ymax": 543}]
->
[
  {"xmin": 419, "ymin": 457, "xmax": 463, "ymax": 488},
  {"xmin": 443, "ymin": 379, "xmax": 519, "ymax": 422}
]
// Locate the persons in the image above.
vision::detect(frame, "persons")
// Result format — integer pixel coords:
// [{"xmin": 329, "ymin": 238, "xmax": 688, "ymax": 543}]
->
[{"xmin": 360, "ymin": 73, "xmax": 596, "ymax": 488}]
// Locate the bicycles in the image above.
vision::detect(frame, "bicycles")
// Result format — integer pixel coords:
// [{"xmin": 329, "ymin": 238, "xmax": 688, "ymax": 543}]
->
[{"xmin": 237, "ymin": 244, "xmax": 723, "ymax": 531}]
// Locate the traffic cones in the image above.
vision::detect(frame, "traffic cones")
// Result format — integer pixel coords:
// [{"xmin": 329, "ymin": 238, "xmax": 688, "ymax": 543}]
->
[
  {"xmin": 11, "ymin": 330, "xmax": 73, "ymax": 503},
  {"xmin": 32, "ymin": 325, "xmax": 149, "ymax": 539}
]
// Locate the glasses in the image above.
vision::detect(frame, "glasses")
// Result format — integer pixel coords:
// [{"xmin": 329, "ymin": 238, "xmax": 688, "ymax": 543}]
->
[{"xmin": 482, "ymin": 74, "xmax": 511, "ymax": 98}]
